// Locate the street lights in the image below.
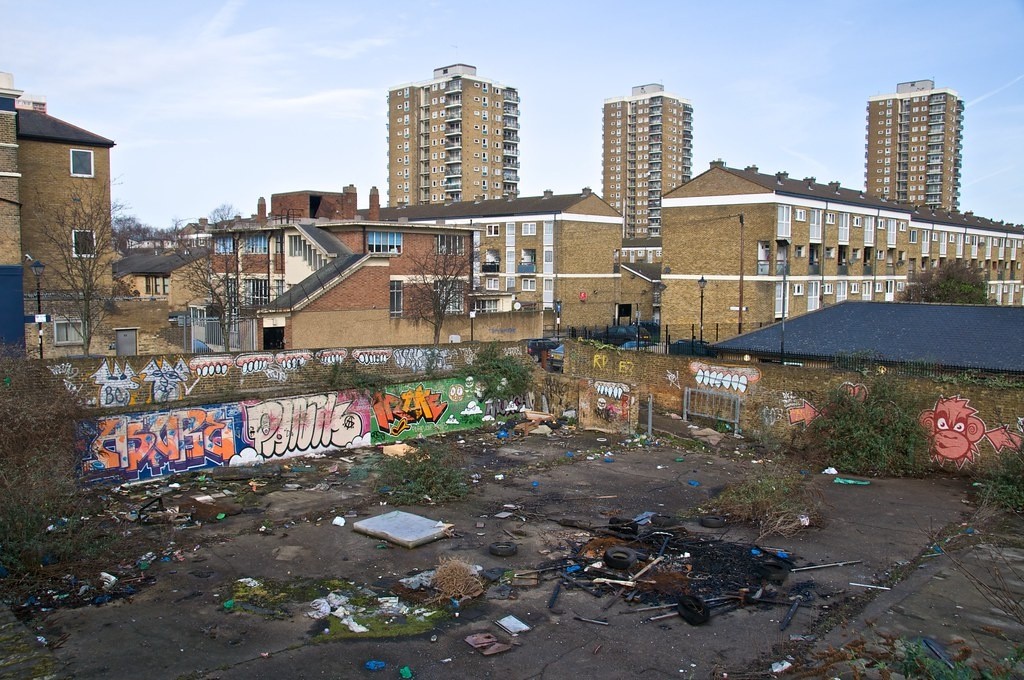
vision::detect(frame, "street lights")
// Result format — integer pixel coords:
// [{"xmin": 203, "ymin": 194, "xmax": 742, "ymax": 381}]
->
[
  {"xmin": 698, "ymin": 276, "xmax": 707, "ymax": 346},
  {"xmin": 30, "ymin": 258, "xmax": 48, "ymax": 363}
]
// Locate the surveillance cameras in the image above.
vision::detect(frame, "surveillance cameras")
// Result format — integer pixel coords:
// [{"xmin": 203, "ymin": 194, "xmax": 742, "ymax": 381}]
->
[{"xmin": 25, "ymin": 253, "xmax": 33, "ymax": 261}]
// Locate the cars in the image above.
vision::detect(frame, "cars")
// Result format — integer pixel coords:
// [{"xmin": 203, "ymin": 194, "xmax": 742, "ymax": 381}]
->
[
  {"xmin": 525, "ymin": 320, "xmax": 660, "ymax": 371},
  {"xmin": 672, "ymin": 339, "xmax": 718, "ymax": 357}
]
